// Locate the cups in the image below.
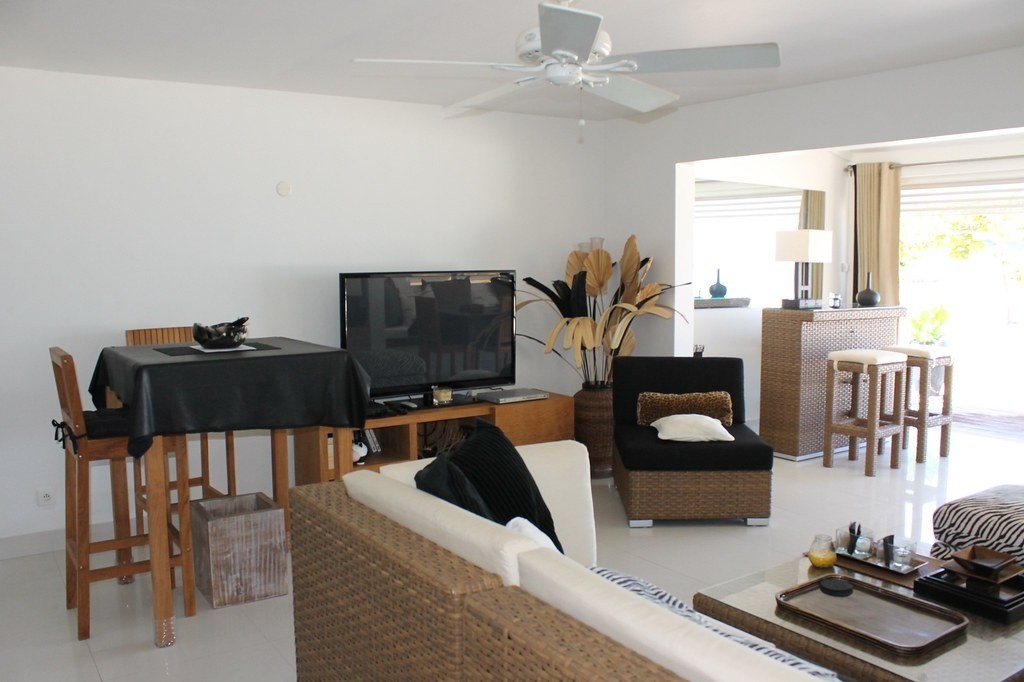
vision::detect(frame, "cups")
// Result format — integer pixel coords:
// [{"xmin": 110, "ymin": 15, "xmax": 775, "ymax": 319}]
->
[{"xmin": 432, "ymin": 386, "xmax": 454, "ymax": 406}]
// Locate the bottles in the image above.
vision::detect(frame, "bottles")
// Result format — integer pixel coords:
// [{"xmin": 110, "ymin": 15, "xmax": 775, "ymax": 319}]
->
[
  {"xmin": 808, "ymin": 533, "xmax": 837, "ymax": 568},
  {"xmin": 834, "ymin": 294, "xmax": 840, "ymax": 308}
]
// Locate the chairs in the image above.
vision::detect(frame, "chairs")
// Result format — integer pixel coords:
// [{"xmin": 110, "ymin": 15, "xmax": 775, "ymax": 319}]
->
[{"xmin": 610, "ymin": 355, "xmax": 775, "ymax": 528}]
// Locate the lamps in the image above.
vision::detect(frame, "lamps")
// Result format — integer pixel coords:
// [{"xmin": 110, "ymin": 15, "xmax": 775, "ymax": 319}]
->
[{"xmin": 771, "ymin": 229, "xmax": 832, "ymax": 309}]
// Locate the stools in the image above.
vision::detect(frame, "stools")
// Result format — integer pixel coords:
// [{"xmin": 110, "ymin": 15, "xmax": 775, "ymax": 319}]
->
[
  {"xmin": 929, "ymin": 483, "xmax": 1023, "ymax": 576},
  {"xmin": 48, "ymin": 326, "xmax": 236, "ymax": 642},
  {"xmin": 824, "ymin": 347, "xmax": 908, "ymax": 478},
  {"xmin": 878, "ymin": 346, "xmax": 955, "ymax": 462}
]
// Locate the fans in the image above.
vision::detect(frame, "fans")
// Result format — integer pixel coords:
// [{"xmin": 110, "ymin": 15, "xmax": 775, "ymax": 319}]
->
[{"xmin": 354, "ymin": 1, "xmax": 780, "ymax": 113}]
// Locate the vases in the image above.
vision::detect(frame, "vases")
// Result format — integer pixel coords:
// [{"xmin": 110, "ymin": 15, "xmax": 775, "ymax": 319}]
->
[{"xmin": 572, "ymin": 382, "xmax": 614, "ymax": 478}]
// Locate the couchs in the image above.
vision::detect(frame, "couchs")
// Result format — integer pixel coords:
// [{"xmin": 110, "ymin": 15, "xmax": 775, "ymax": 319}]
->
[{"xmin": 288, "ymin": 440, "xmax": 848, "ymax": 682}]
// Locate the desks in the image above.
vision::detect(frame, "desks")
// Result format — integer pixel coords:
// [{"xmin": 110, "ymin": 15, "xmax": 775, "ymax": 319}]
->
[{"xmin": 86, "ymin": 337, "xmax": 371, "ymax": 619}]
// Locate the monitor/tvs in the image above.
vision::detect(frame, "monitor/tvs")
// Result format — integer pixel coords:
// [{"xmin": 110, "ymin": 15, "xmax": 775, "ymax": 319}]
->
[{"xmin": 337, "ymin": 269, "xmax": 517, "ymax": 411}]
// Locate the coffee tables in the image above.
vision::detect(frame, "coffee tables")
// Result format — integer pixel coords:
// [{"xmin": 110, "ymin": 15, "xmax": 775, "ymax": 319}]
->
[{"xmin": 693, "ymin": 537, "xmax": 1024, "ymax": 682}]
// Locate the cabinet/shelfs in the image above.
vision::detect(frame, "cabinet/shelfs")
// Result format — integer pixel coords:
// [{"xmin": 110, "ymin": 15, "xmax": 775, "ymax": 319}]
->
[{"xmin": 295, "ymin": 386, "xmax": 576, "ymax": 484}]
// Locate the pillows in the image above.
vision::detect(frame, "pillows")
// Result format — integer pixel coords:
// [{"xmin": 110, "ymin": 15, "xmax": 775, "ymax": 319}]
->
[
  {"xmin": 638, "ymin": 390, "xmax": 733, "ymax": 426},
  {"xmin": 649, "ymin": 414, "xmax": 736, "ymax": 442}
]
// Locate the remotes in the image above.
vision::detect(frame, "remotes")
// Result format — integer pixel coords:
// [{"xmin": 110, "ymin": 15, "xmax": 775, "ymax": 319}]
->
[{"xmin": 400, "ymin": 401, "xmax": 418, "ymax": 409}]
